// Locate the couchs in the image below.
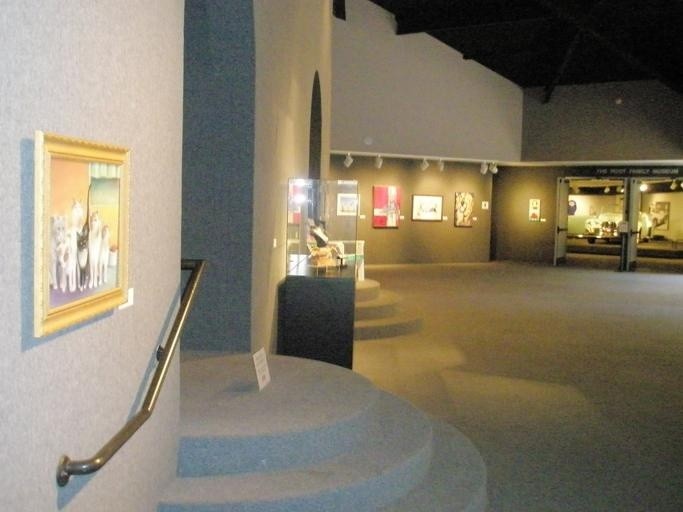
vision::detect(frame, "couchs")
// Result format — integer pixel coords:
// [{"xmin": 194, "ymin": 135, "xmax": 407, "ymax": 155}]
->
[{"xmin": 308, "ymin": 238, "xmax": 341, "ymax": 276}]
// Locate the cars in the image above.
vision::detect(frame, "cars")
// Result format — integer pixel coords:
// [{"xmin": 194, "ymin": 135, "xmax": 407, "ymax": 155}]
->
[{"xmin": 582, "ymin": 201, "xmax": 654, "ymax": 245}]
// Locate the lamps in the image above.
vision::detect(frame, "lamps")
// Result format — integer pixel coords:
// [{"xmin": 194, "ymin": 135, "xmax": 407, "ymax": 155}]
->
[
  {"xmin": 418, "ymin": 158, "xmax": 429, "ymax": 171},
  {"xmin": 489, "ymin": 162, "xmax": 498, "ymax": 174},
  {"xmin": 616, "ymin": 183, "xmax": 622, "ymax": 192},
  {"xmin": 437, "ymin": 158, "xmax": 445, "ymax": 172},
  {"xmin": 572, "ymin": 184, "xmax": 579, "ymax": 193},
  {"xmin": 670, "ymin": 179, "xmax": 677, "ymax": 191},
  {"xmin": 374, "ymin": 154, "xmax": 383, "ymax": 168},
  {"xmin": 480, "ymin": 161, "xmax": 488, "ymax": 175},
  {"xmin": 343, "ymin": 153, "xmax": 353, "ymax": 166},
  {"xmin": 604, "ymin": 185, "xmax": 610, "ymax": 193}
]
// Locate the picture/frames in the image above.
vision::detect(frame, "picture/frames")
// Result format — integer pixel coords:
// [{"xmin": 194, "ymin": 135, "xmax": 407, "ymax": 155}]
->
[
  {"xmin": 655, "ymin": 202, "xmax": 670, "ymax": 230},
  {"xmin": 455, "ymin": 193, "xmax": 475, "ymax": 227},
  {"xmin": 411, "ymin": 194, "xmax": 444, "ymax": 222},
  {"xmin": 337, "ymin": 180, "xmax": 360, "ymax": 216},
  {"xmin": 33, "ymin": 129, "xmax": 130, "ymax": 340},
  {"xmin": 373, "ymin": 185, "xmax": 401, "ymax": 227}
]
